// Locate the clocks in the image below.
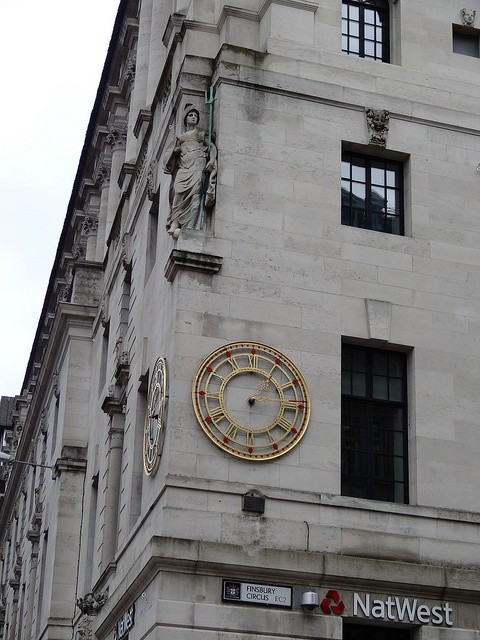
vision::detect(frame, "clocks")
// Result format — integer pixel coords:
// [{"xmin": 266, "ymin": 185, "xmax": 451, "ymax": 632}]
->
[
  {"xmin": 190, "ymin": 339, "xmax": 311, "ymax": 463},
  {"xmin": 143, "ymin": 357, "xmax": 170, "ymax": 478}
]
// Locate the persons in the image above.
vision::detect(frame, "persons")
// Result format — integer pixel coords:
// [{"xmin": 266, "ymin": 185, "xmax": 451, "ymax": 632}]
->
[
  {"xmin": 162, "ymin": 105, "xmax": 218, "ymax": 234},
  {"xmin": 461, "ymin": 8, "xmax": 476, "ymax": 28},
  {"xmin": 366, "ymin": 107, "xmax": 390, "ymax": 132}
]
